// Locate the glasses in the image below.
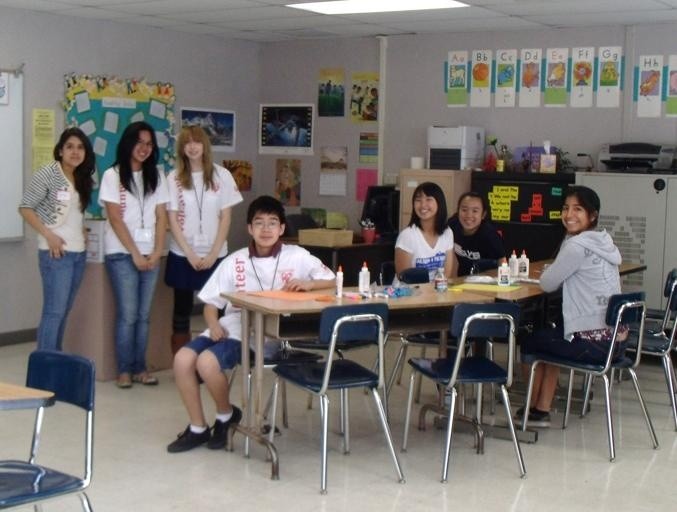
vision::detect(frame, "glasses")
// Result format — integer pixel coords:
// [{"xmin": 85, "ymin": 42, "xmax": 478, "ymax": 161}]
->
[{"xmin": 253, "ymin": 220, "xmax": 281, "ymax": 229}]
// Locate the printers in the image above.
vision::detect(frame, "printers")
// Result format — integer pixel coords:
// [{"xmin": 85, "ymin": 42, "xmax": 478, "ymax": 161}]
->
[{"xmin": 597, "ymin": 142, "xmax": 677, "ymax": 173}]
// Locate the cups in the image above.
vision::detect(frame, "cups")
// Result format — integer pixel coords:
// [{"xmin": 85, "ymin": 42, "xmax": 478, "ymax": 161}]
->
[
  {"xmin": 495, "ymin": 159, "xmax": 504, "ymax": 171},
  {"xmin": 411, "ymin": 156, "xmax": 426, "ymax": 169},
  {"xmin": 361, "ymin": 226, "xmax": 375, "ymax": 243}
]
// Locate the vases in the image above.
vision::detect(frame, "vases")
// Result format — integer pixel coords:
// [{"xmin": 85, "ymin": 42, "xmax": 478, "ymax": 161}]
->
[{"xmin": 496, "ymin": 160, "xmax": 504, "ymax": 172}]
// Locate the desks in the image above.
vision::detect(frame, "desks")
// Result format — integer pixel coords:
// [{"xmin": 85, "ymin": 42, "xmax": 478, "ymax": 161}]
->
[
  {"xmin": 219, "ymin": 262, "xmax": 649, "ymax": 480},
  {"xmin": 309, "ymin": 239, "xmax": 396, "ymax": 275},
  {"xmin": 0, "ymin": 383, "xmax": 55, "ymax": 410}
]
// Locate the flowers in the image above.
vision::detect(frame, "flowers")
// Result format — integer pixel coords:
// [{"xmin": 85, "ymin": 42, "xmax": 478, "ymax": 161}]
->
[{"xmin": 488, "ymin": 136, "xmax": 502, "ymax": 160}]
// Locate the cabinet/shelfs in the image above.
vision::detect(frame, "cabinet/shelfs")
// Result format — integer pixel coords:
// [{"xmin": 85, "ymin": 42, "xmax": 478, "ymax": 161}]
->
[
  {"xmin": 471, "ymin": 180, "xmax": 568, "ymax": 260},
  {"xmin": 400, "ymin": 170, "xmax": 471, "ymax": 230},
  {"xmin": 575, "ymin": 175, "xmax": 677, "ymax": 311}
]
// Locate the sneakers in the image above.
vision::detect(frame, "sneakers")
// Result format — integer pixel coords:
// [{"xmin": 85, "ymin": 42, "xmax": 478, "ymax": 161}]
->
[
  {"xmin": 168, "ymin": 424, "xmax": 211, "ymax": 452},
  {"xmin": 515, "ymin": 405, "xmax": 551, "ymax": 428},
  {"xmin": 212, "ymin": 406, "xmax": 242, "ymax": 450},
  {"xmin": 118, "ymin": 372, "xmax": 131, "ymax": 387},
  {"xmin": 133, "ymin": 371, "xmax": 158, "ymax": 384}
]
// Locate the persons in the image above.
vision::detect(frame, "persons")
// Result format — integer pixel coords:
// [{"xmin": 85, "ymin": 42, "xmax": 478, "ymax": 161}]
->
[
  {"xmin": 165, "ymin": 125, "xmax": 245, "ymax": 384},
  {"xmin": 265, "ymin": 114, "xmax": 308, "ymax": 148},
  {"xmin": 97, "ymin": 121, "xmax": 170, "ymax": 387},
  {"xmin": 351, "ymin": 83, "xmax": 379, "ymax": 119},
  {"xmin": 319, "ymin": 79, "xmax": 344, "ymax": 115},
  {"xmin": 512, "ymin": 185, "xmax": 629, "ymax": 427},
  {"xmin": 18, "ymin": 127, "xmax": 95, "ymax": 353},
  {"xmin": 167, "ymin": 196, "xmax": 337, "ymax": 453},
  {"xmin": 394, "ymin": 182, "xmax": 469, "ymax": 420},
  {"xmin": 447, "ymin": 192, "xmax": 505, "ymax": 359}
]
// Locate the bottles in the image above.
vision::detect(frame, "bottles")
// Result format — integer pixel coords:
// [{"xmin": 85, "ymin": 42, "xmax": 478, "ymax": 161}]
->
[
  {"xmin": 334, "ymin": 264, "xmax": 345, "ymax": 298},
  {"xmin": 509, "ymin": 249, "xmax": 519, "ymax": 276},
  {"xmin": 518, "ymin": 248, "xmax": 530, "ymax": 277},
  {"xmin": 358, "ymin": 262, "xmax": 371, "ymax": 293},
  {"xmin": 434, "ymin": 267, "xmax": 448, "ymax": 293},
  {"xmin": 497, "ymin": 256, "xmax": 510, "ymax": 286},
  {"xmin": 499, "ymin": 144, "xmax": 511, "ymax": 170}
]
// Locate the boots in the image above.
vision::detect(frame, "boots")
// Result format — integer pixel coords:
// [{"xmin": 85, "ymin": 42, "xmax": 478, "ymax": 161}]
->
[{"xmin": 172, "ymin": 333, "xmax": 203, "ymax": 384}]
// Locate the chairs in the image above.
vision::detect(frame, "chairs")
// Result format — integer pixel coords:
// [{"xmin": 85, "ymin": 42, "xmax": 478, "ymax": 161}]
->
[
  {"xmin": 286, "ymin": 214, "xmax": 318, "ymax": 238},
  {"xmin": 624, "ymin": 269, "xmax": 677, "ymax": 437},
  {"xmin": 400, "ymin": 299, "xmax": 534, "ymax": 487},
  {"xmin": 265, "ymin": 303, "xmax": 411, "ymax": 494},
  {"xmin": 526, "ymin": 291, "xmax": 659, "ymax": 463},
  {"xmin": 0, "ymin": 350, "xmax": 96, "ymax": 512}
]
